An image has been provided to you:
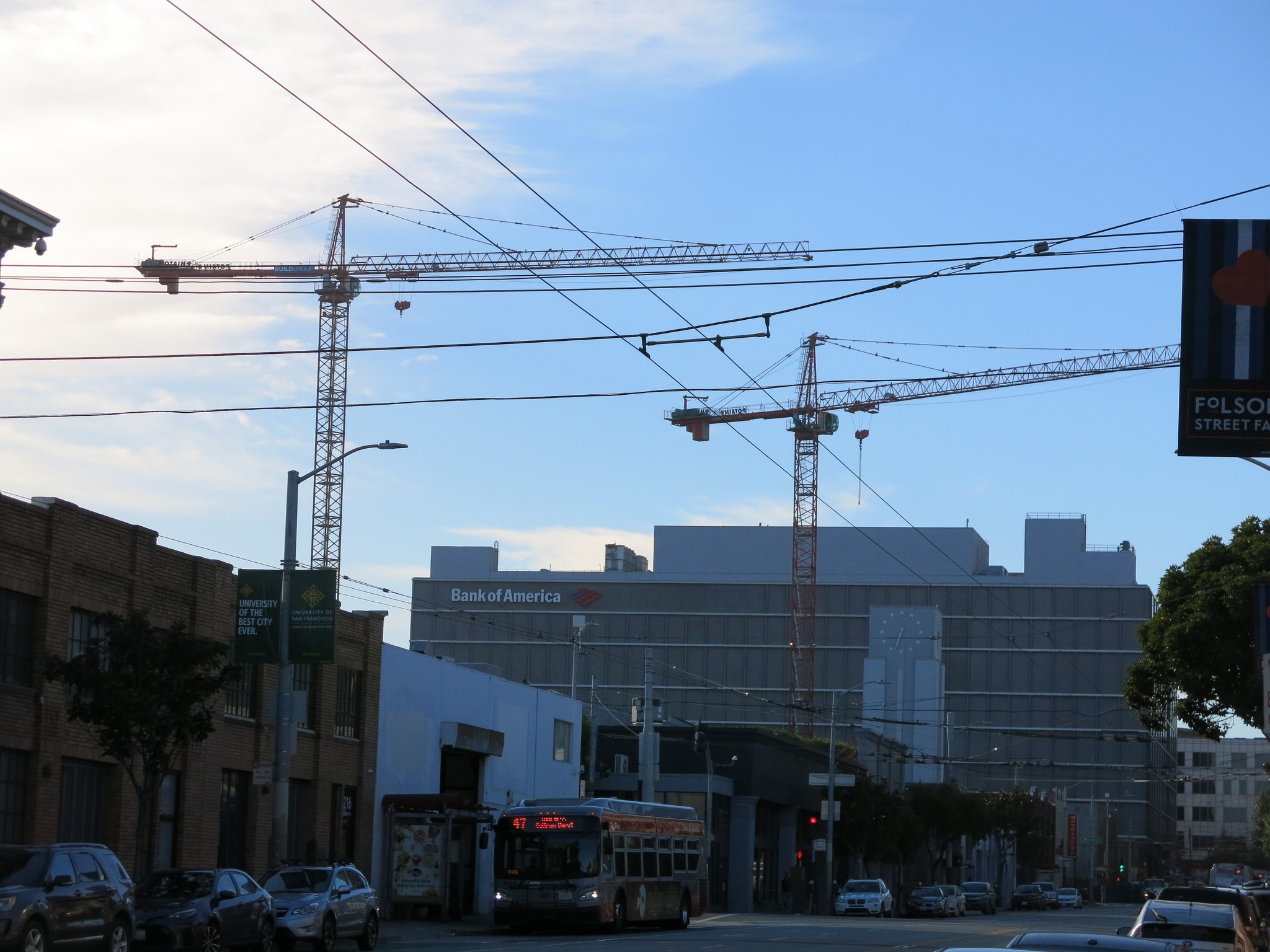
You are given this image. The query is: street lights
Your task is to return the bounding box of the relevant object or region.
[1060,778,1091,885]
[824,677,892,917]
[1103,794,1137,904]
[1013,758,1054,885]
[570,619,601,697]
[268,439,411,874]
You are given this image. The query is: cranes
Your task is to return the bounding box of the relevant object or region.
[667,339,1181,730]
[136,196,815,603]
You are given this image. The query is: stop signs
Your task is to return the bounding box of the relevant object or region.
[1060,857,1071,862]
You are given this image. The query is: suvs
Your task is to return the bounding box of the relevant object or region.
[1155,878,1270,952]
[834,877,894,915]
[1,843,138,952]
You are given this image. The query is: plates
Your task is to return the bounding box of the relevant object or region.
[422,853,434,866]
[413,829,427,843]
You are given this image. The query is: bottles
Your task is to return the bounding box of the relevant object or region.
[434,861,439,876]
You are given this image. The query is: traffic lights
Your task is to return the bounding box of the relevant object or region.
[810,812,819,831]
[1116,865,1124,883]
[796,850,808,861]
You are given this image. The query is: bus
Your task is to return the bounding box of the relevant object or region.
[478,797,709,932]
[256,859,380,952]
[1209,863,1254,887]
[1140,878,1173,903]
[1251,869,1270,883]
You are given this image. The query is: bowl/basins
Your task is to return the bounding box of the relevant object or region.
[412,868,422,876]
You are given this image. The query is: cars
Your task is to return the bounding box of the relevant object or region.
[1115,898,1265,952]
[135,869,277,952]
[932,883,967,918]
[1011,880,1088,913]
[1005,931,1187,952]
[956,881,996,916]
[905,886,948,918]
